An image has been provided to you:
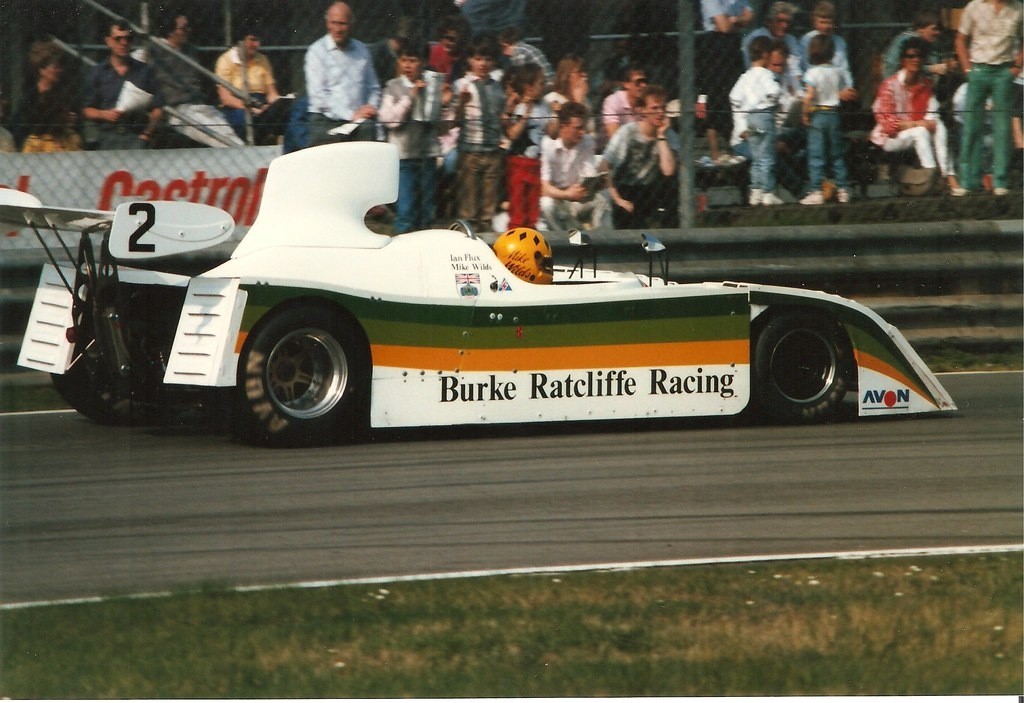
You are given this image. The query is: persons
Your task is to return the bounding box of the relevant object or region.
[493,227,553,284]
[1,0,1024,234]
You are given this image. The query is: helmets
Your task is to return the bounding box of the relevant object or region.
[493,226,554,284]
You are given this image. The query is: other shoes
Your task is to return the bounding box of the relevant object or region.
[992,187,1012,196]
[748,188,767,206]
[714,153,747,164]
[762,193,784,206]
[949,187,971,196]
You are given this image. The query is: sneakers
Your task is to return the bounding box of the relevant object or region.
[798,191,824,205]
[837,189,849,203]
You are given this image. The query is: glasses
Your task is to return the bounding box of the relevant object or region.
[439,33,459,43]
[107,34,133,43]
[628,77,649,86]
[904,53,924,59]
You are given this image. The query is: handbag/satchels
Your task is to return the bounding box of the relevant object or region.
[895,164,938,196]
